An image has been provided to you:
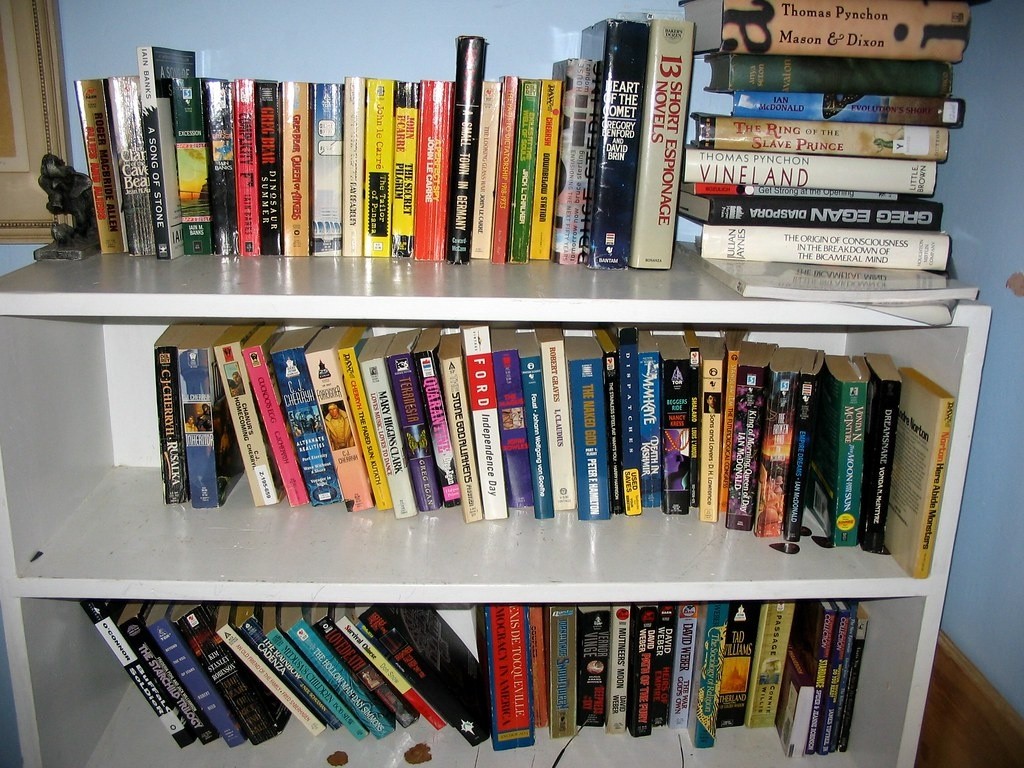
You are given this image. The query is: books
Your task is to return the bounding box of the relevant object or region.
[76,1,981,306]
[153,323,956,580]
[78,600,872,759]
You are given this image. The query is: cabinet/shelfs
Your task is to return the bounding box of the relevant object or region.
[0,260,996,768]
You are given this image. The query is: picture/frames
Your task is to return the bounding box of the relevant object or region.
[0,0,75,245]
